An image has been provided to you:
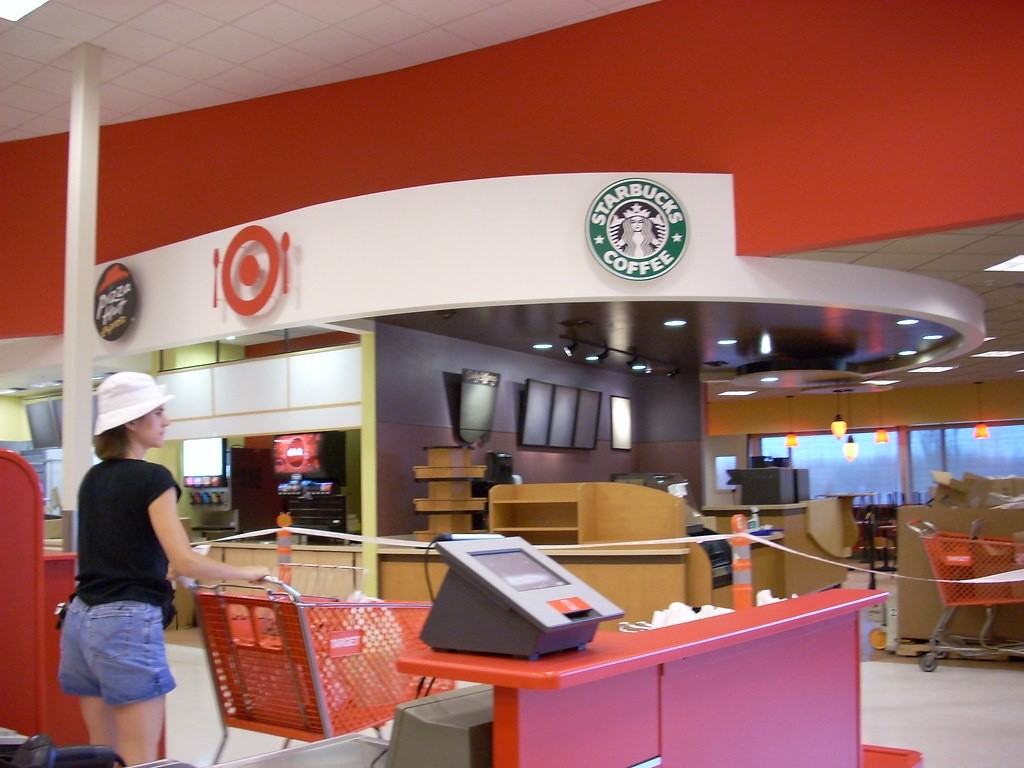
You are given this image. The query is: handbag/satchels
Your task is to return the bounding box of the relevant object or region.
[162,586,178,631]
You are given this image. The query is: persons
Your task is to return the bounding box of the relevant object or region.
[60,371,271,768]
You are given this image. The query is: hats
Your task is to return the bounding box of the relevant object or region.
[93,371,175,436]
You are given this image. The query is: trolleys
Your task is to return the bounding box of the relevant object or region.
[904,519,1023,671]
[190,562,454,767]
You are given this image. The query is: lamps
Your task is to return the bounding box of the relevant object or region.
[972,381,990,440]
[844,389,859,463]
[585,341,610,362]
[625,355,646,370]
[784,395,798,447]
[563,330,580,357]
[666,369,678,378]
[876,391,890,444]
[831,390,847,440]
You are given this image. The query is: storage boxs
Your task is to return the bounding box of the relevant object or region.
[931,470,1024,509]
